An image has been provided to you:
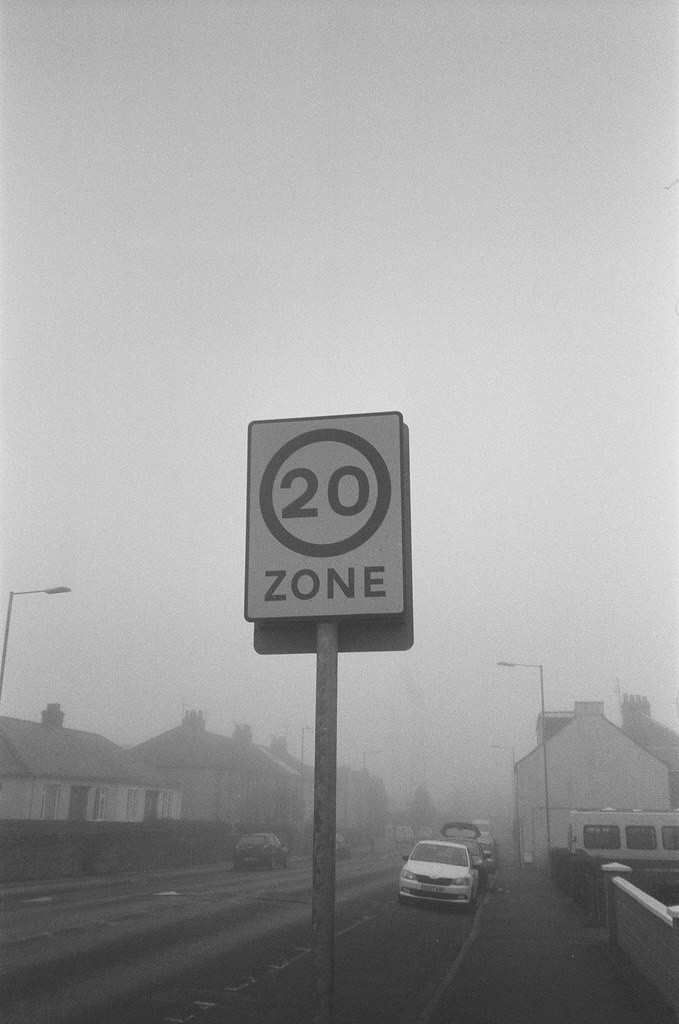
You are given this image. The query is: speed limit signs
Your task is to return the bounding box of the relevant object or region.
[259,425,395,558]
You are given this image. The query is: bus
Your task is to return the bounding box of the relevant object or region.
[566,807,679,868]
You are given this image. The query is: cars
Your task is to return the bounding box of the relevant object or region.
[231,832,290,873]
[429,818,498,878]
[396,839,479,911]
[305,832,350,861]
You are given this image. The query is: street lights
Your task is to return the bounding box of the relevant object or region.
[490,745,522,872]
[1,586,73,703]
[496,661,555,877]
